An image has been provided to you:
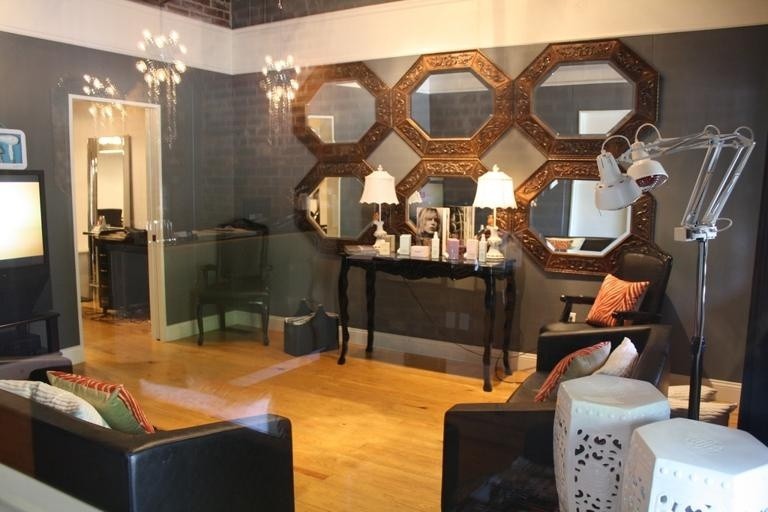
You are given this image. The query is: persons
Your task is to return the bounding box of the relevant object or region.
[413,206,438,238]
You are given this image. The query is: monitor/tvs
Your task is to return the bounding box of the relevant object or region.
[0,170,50,269]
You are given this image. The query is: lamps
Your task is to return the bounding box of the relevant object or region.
[472,166,519,258]
[593,112,757,417]
[360,165,399,245]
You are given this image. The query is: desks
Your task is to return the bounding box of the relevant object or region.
[332,246,518,391]
[92,230,148,317]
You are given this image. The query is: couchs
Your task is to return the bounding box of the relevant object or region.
[0,355,295,511]
[437,322,673,512]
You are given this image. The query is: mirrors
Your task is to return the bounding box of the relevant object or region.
[90,136,129,233]
[513,38,658,160]
[390,159,510,249]
[291,163,389,254]
[510,159,656,273]
[291,60,393,162]
[390,49,514,158]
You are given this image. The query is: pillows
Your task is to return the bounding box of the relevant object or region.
[531,336,610,400]
[0,376,114,430]
[45,367,154,436]
[590,336,638,379]
[585,267,649,326]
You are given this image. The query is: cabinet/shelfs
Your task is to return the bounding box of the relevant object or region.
[0,310,73,382]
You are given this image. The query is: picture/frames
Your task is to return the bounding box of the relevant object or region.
[0,127,28,171]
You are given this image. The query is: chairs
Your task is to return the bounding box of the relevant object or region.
[192,213,271,347]
[536,234,673,335]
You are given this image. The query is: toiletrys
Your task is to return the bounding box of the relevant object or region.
[479,233,487,263]
[478,234,488,262]
[465,239,479,260]
